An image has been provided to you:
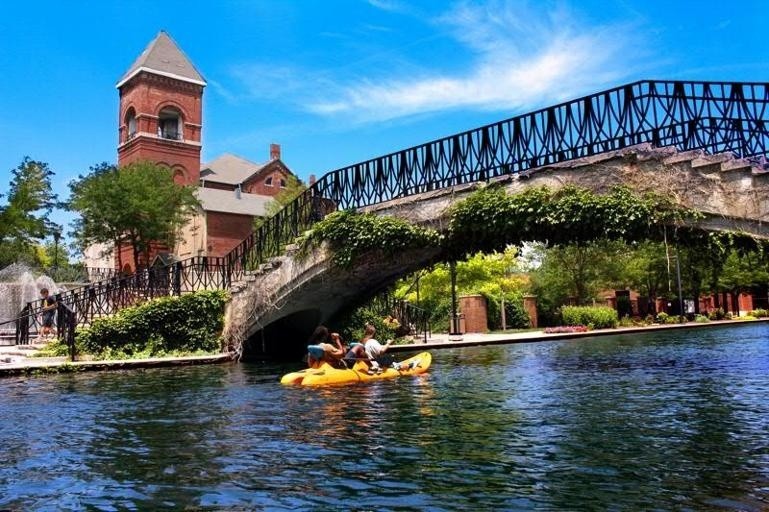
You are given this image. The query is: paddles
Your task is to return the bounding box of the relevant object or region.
[343,357,393,364]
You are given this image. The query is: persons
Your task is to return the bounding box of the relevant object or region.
[34,288,58,341]
[360,324,420,370]
[306,325,382,375]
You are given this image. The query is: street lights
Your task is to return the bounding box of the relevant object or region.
[53,227,62,271]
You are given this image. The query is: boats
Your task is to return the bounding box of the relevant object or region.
[279,350,434,388]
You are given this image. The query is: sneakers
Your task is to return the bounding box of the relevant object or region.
[412,359,421,369]
[369,367,379,371]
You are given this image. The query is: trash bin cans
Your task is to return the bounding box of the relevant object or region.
[449,313,466,335]
